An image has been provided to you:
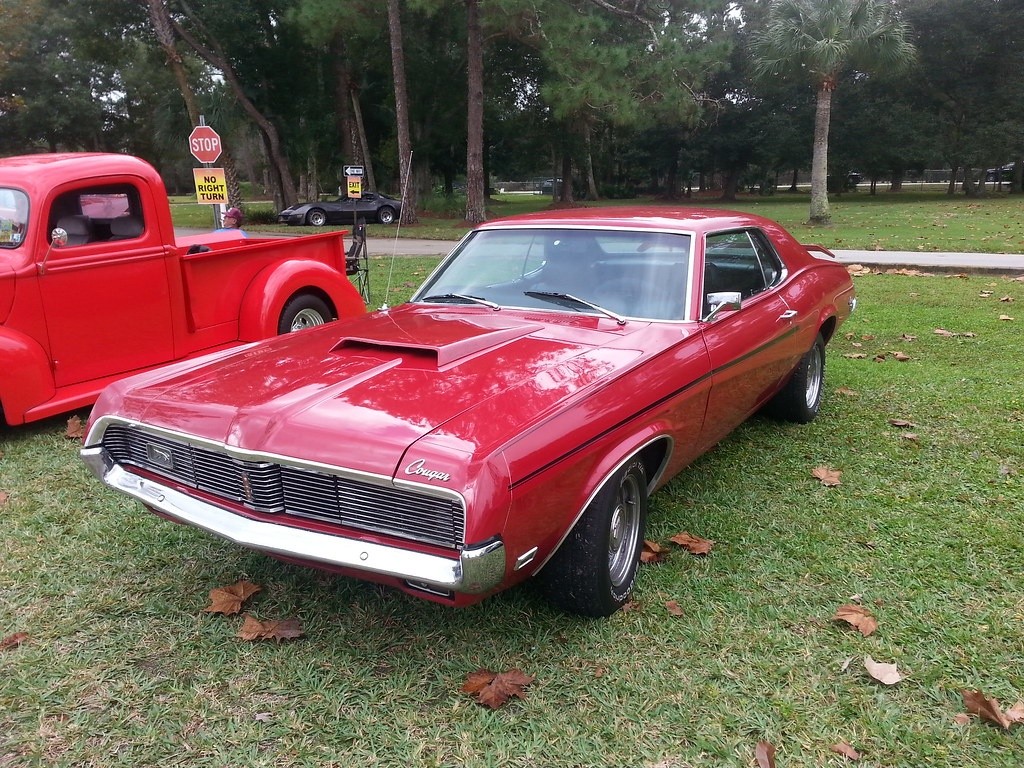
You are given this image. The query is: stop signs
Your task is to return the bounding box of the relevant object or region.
[188,125,223,164]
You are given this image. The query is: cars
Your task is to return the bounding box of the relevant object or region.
[984,161,1016,182]
[79,204,857,620]
[276,190,402,228]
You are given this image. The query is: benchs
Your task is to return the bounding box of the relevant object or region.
[602,259,773,301]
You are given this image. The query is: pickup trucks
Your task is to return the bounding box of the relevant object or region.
[1,151,367,435]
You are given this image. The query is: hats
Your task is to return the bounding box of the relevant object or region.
[222,208,242,219]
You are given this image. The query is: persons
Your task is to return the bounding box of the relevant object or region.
[211,207,248,237]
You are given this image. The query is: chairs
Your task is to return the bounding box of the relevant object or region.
[345,217,371,306]
[53,215,93,248]
[108,217,144,241]
